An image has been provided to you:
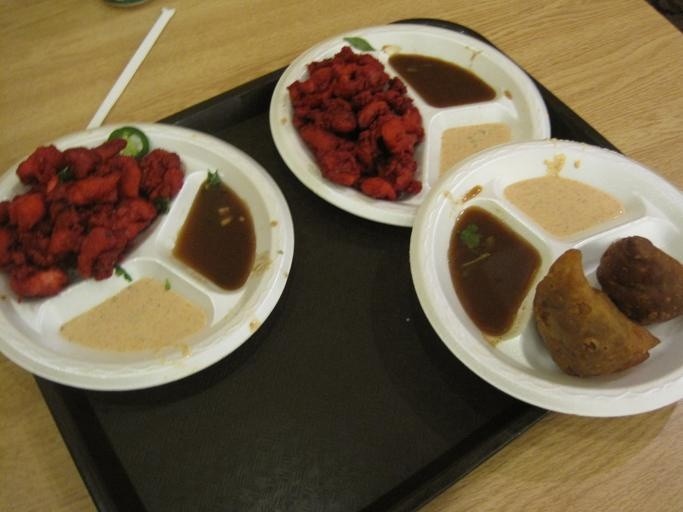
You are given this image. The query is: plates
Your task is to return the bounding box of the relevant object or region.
[0,115,294,392]
[24,12,657,512]
[418,136,683,409]
[277,15,543,226]
